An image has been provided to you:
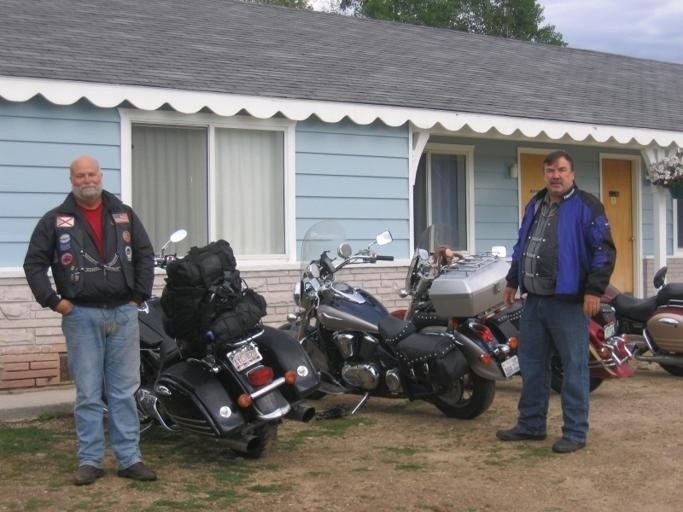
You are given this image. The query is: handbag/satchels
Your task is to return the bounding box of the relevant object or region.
[160,239,265,348]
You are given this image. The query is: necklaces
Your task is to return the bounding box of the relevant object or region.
[540,203,559,217]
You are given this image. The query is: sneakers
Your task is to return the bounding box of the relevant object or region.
[117,463,157,481]
[496,428,546,441]
[554,438,587,454]
[75,463,101,484]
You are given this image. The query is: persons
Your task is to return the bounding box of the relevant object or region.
[24,156,157,486]
[495,151,616,453]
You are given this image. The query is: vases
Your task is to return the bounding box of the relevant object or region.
[667,180,682,199]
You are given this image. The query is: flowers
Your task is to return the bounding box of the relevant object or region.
[646,146,683,184]
